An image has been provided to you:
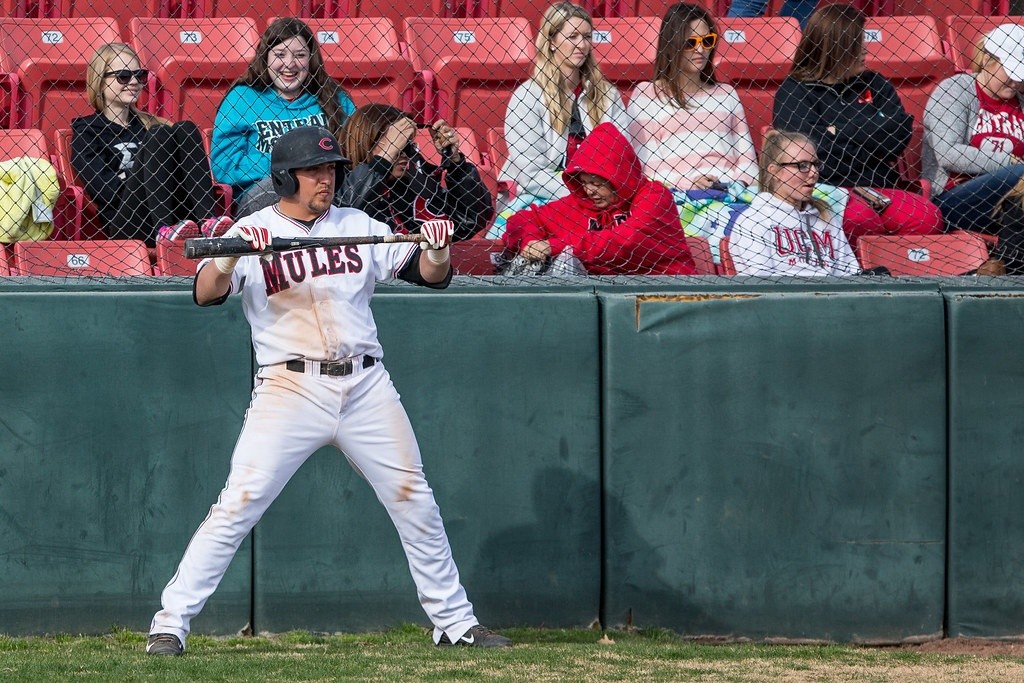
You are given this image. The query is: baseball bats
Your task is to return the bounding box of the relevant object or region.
[184,234,426,259]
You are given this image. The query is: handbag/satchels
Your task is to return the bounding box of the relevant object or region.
[503,246,588,276]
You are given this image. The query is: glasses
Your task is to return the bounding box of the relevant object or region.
[776,158,824,174]
[102,69,149,86]
[682,33,717,52]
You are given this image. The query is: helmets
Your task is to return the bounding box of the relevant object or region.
[272,126,350,198]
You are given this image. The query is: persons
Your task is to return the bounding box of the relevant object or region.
[328,104,493,241]
[496,4,630,200]
[627,4,759,191]
[920,24,1024,273]
[506,122,698,275]
[146,125,511,653]
[728,130,890,276]
[211,17,357,221]
[725,0,820,31]
[773,4,941,235]
[72,43,236,248]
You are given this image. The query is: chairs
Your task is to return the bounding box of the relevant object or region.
[0,0,1024,276]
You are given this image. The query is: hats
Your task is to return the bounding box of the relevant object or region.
[984,23,1024,81]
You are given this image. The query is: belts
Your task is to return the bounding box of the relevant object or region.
[286,354,379,377]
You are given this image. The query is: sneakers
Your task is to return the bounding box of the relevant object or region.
[155,220,198,244]
[199,217,234,237]
[146,633,184,657]
[434,620,510,647]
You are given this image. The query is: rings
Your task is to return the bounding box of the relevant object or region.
[448,132,452,137]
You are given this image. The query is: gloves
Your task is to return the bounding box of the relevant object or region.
[419,218,455,266]
[215,228,273,275]
